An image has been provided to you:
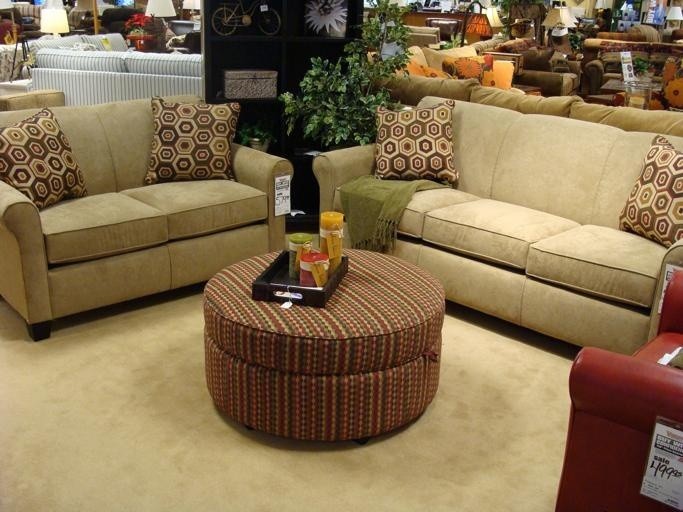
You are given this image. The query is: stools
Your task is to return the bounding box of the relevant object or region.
[204,246,447,446]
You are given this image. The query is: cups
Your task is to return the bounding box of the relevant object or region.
[623,80,652,112]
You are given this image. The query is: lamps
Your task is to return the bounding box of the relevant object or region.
[144,0,177,17]
[459,0,494,46]
[665,7,683,29]
[40,8,70,36]
[182,0,201,21]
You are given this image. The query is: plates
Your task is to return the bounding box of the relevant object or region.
[250,249,349,309]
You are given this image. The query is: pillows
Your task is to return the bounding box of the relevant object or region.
[519,49,555,72]
[407,58,458,81]
[0,108,86,210]
[442,54,496,88]
[494,39,529,53]
[619,133,683,248]
[145,95,240,187]
[372,101,456,186]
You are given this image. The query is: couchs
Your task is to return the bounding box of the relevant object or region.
[554,269,683,512]
[311,97,683,358]
[30,47,207,107]
[584,48,667,97]
[0,93,295,343]
[471,38,582,96]
[408,45,515,90]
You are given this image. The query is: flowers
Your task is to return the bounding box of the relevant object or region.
[650,57,683,112]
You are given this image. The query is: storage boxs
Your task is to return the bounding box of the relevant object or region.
[223,69,278,98]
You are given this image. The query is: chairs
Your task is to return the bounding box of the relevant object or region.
[438,45,477,57]
